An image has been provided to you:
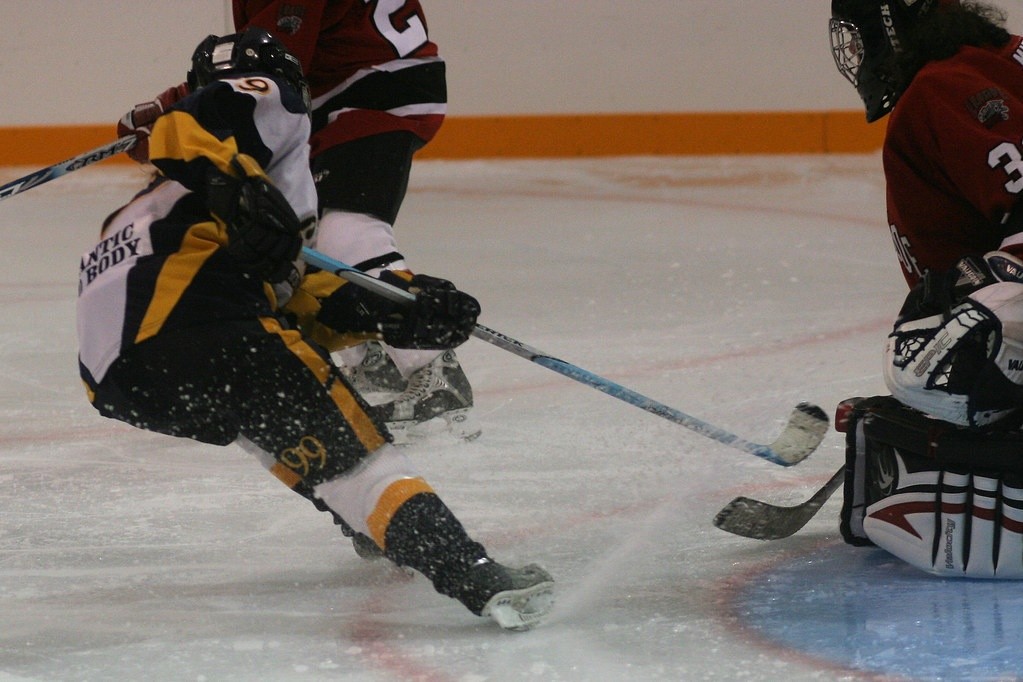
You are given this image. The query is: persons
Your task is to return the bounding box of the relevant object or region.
[73,28,558,631]
[826,0,1023,584]
[233,0,484,448]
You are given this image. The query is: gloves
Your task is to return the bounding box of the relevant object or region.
[207,154,301,286]
[372,269,482,350]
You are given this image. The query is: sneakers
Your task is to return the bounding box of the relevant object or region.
[337,342,409,403]
[457,555,560,631]
[373,349,482,449]
[350,534,409,581]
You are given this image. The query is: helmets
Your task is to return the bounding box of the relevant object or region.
[185,24,303,112]
[830,0,942,123]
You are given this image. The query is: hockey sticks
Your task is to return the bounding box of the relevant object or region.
[296,246,830,468]
[0,135,137,201]
[711,463,846,541]
